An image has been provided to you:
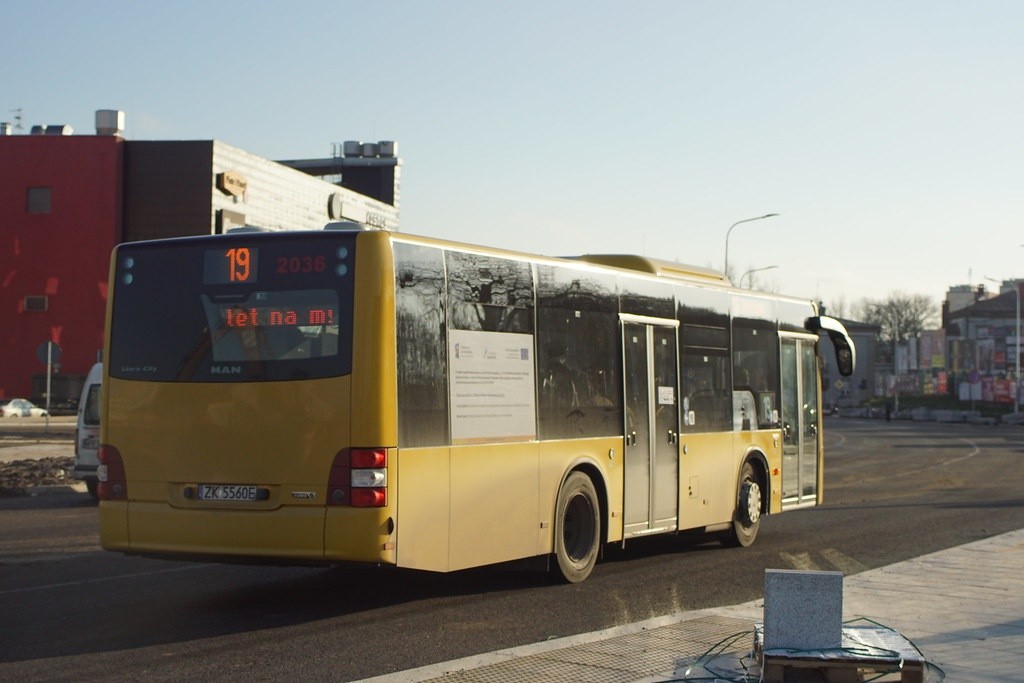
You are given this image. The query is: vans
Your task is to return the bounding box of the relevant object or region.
[72,362,104,501]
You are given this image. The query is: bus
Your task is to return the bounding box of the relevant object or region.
[99,221,858,582]
